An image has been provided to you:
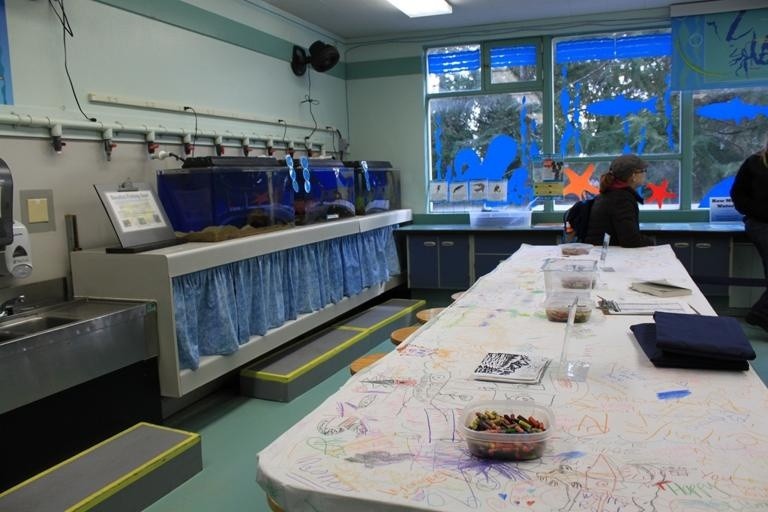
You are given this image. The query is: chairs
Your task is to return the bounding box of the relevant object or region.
[563,197,594,241]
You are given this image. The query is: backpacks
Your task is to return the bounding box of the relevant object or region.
[560,195,598,243]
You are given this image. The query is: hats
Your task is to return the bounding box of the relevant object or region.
[609,154,650,176]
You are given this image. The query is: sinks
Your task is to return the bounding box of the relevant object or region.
[0,316,80,346]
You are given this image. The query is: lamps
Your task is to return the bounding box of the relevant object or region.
[290,38,343,78]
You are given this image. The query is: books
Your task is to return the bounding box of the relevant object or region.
[630,278,693,297]
[473,352,553,384]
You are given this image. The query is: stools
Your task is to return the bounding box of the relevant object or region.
[349,288,469,376]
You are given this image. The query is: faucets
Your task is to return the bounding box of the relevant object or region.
[0,294,25,314]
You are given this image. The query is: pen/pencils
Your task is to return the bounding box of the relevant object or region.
[467,409,547,461]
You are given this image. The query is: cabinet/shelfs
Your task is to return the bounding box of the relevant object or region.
[401,233,745,294]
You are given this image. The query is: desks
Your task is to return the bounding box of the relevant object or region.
[256,239,765,510]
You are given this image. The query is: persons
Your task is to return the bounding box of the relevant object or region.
[730,132,768,332]
[544,159,553,169]
[584,154,656,248]
